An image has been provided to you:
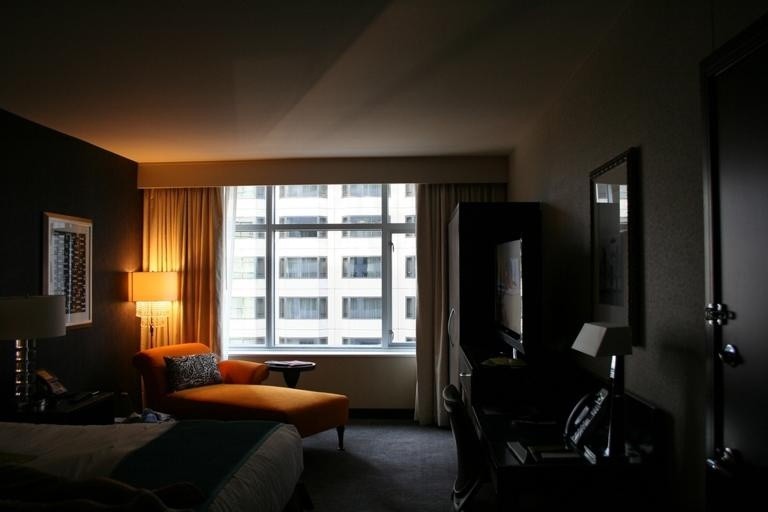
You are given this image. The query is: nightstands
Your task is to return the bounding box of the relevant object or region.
[10,389,117,423]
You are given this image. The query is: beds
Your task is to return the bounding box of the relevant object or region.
[1,421,310,511]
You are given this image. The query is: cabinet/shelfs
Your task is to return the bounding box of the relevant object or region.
[447,201,542,395]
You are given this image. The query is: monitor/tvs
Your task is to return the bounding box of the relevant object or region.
[486,236,535,354]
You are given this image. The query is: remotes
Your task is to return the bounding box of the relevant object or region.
[67,390,93,404]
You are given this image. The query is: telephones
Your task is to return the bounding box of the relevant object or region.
[564,388,611,450]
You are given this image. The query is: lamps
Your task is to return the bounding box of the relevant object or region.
[0,291,67,409]
[126,270,180,348]
[570,322,634,458]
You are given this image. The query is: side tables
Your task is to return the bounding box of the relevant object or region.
[265,360,317,387]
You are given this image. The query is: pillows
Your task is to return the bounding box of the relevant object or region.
[162,352,223,392]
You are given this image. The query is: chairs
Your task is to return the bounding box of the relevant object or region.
[442,385,490,512]
[132,341,348,451]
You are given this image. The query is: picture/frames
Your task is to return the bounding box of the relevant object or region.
[43,212,94,332]
[589,146,643,347]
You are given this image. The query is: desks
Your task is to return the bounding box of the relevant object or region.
[470,406,641,497]
